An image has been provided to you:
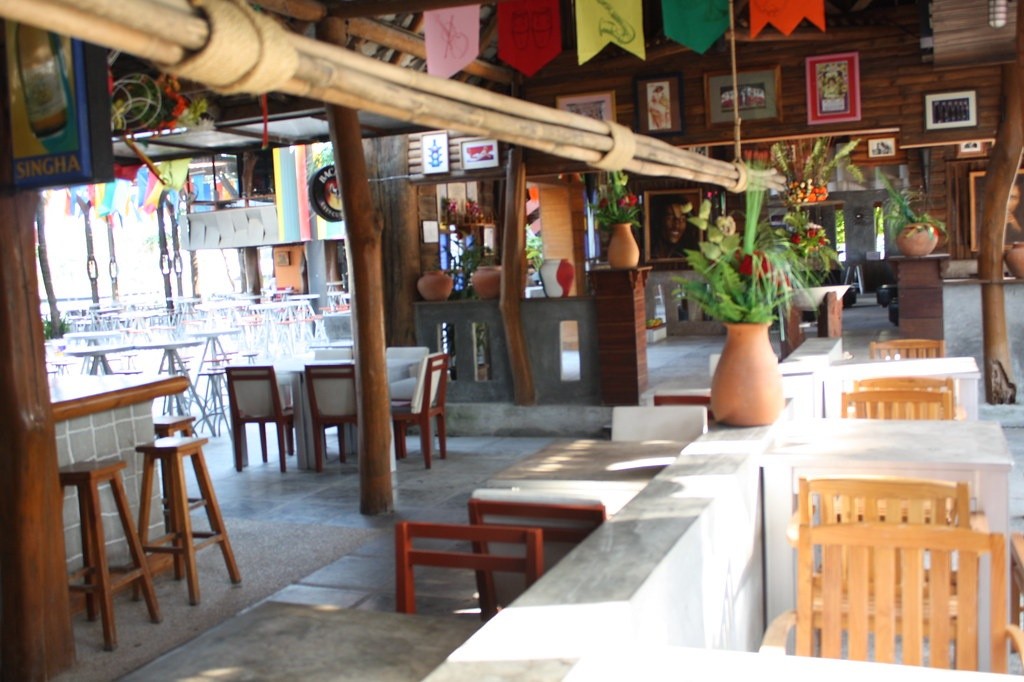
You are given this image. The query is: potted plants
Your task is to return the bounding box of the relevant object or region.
[869,168,951,257]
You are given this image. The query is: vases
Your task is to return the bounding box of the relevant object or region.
[711,320,789,427]
[607,222,639,270]
[792,285,852,312]
[1005,241,1024,280]
[472,265,503,299]
[417,270,454,302]
[539,257,576,299]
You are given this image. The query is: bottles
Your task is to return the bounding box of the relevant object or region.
[16,22,68,138]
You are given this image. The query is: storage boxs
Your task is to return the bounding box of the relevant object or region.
[646,325,667,344]
[866,252,880,261]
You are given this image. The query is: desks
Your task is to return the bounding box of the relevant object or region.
[114,600,484,682]
[271,356,424,469]
[48,376,187,616]
[469,432,694,517]
[117,308,157,343]
[286,293,321,318]
[188,327,242,438]
[63,343,135,377]
[193,299,252,329]
[818,354,981,423]
[134,340,216,439]
[251,300,310,357]
[62,330,121,376]
[760,415,1018,676]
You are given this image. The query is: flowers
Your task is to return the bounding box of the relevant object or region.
[113,67,205,132]
[585,178,835,328]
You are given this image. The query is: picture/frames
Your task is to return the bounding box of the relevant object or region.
[866,135,898,161]
[703,63,785,131]
[641,188,707,267]
[805,50,861,126]
[922,86,984,135]
[420,130,451,176]
[275,251,291,267]
[970,169,1024,253]
[554,89,619,126]
[633,71,687,138]
[459,136,502,172]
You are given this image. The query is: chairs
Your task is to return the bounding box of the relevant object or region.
[225,347,727,620]
[757,339,1024,676]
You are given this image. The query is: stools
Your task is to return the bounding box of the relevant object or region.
[844,261,866,296]
[44,280,353,654]
